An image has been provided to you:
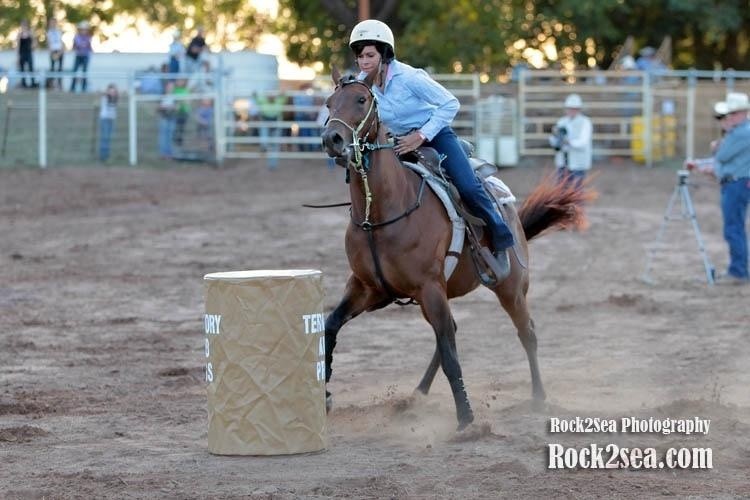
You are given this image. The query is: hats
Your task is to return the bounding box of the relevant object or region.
[714,93,749,119]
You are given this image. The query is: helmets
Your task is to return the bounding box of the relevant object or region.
[565,94,582,110]
[348,19,395,58]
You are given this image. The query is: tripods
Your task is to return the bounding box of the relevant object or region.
[642,171,717,289]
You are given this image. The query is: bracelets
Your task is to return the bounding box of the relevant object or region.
[420,133,426,140]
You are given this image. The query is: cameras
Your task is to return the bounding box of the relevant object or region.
[558,126,569,137]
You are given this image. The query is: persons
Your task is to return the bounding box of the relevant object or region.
[348,20,516,278]
[45,18,66,90]
[548,94,593,190]
[155,37,216,159]
[15,20,40,87]
[99,83,118,164]
[252,83,324,169]
[710,92,750,281]
[69,21,92,92]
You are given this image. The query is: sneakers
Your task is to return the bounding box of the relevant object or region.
[716,272,736,283]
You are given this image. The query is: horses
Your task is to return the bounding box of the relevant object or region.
[320,62,604,440]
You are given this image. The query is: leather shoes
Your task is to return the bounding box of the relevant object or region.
[488,250,511,279]
